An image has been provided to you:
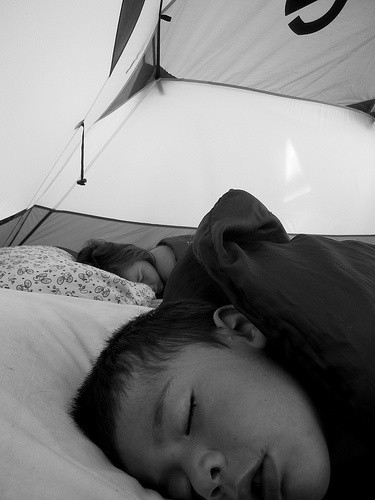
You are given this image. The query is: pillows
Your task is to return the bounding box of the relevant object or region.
[0,244,158,310]
[0,287,164,500]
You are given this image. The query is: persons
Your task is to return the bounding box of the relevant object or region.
[63,284,332,500]
[74,232,191,296]
